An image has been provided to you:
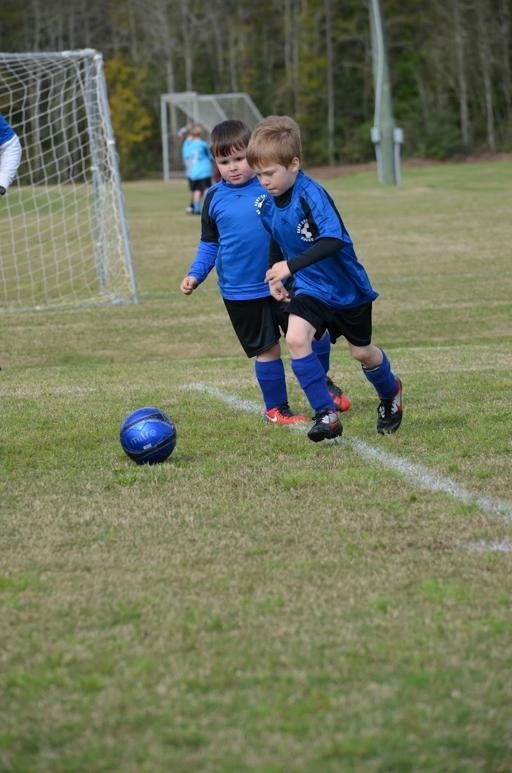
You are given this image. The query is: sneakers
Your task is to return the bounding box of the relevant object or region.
[377,377,402,435]
[188,206,201,215]
[264,378,349,442]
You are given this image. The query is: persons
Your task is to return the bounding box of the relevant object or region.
[177,117,222,214]
[246,116,404,439]
[180,120,350,425]
[0,112,22,199]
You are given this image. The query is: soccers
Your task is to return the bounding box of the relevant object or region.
[119,408,175,465]
